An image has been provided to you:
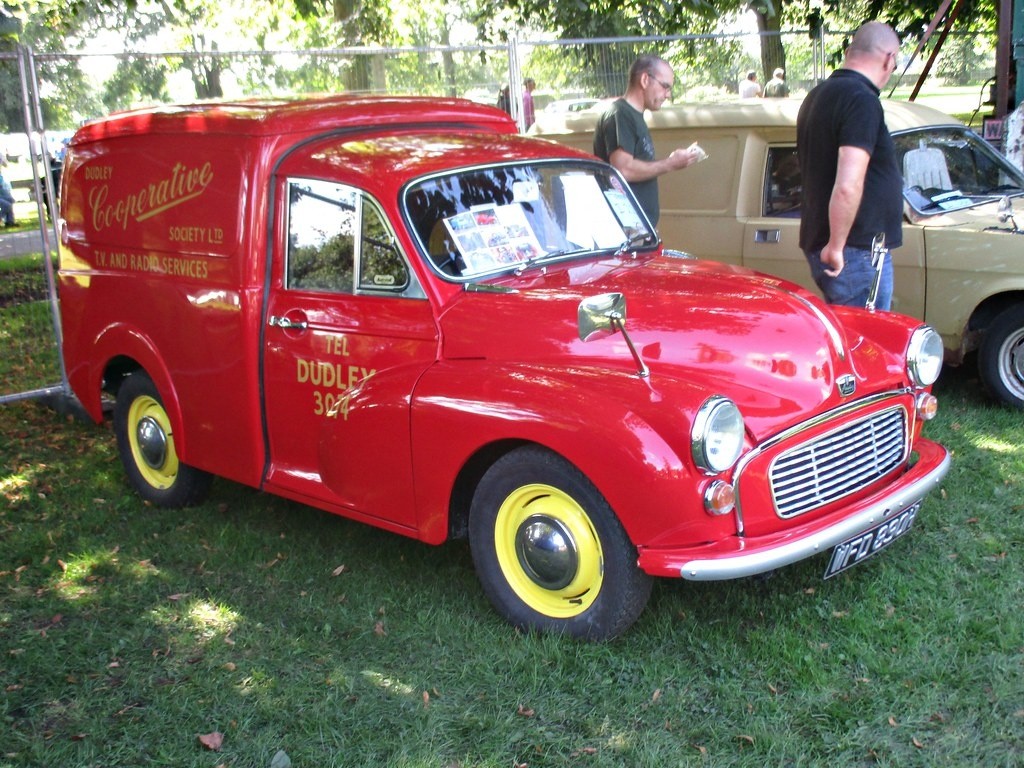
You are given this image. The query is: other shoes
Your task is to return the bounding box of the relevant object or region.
[5,222,20,228]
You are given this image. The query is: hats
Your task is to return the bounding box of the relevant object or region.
[774,68,784,77]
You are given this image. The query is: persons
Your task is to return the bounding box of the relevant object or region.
[738,69,760,98]
[593,55,700,232]
[797,21,904,312]
[494,77,536,133]
[761,67,791,98]
[0,154,21,227]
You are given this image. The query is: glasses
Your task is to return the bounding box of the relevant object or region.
[888,53,898,73]
[648,73,670,90]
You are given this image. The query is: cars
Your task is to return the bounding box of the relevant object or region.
[51,101,951,652]
[524,95,1024,411]
